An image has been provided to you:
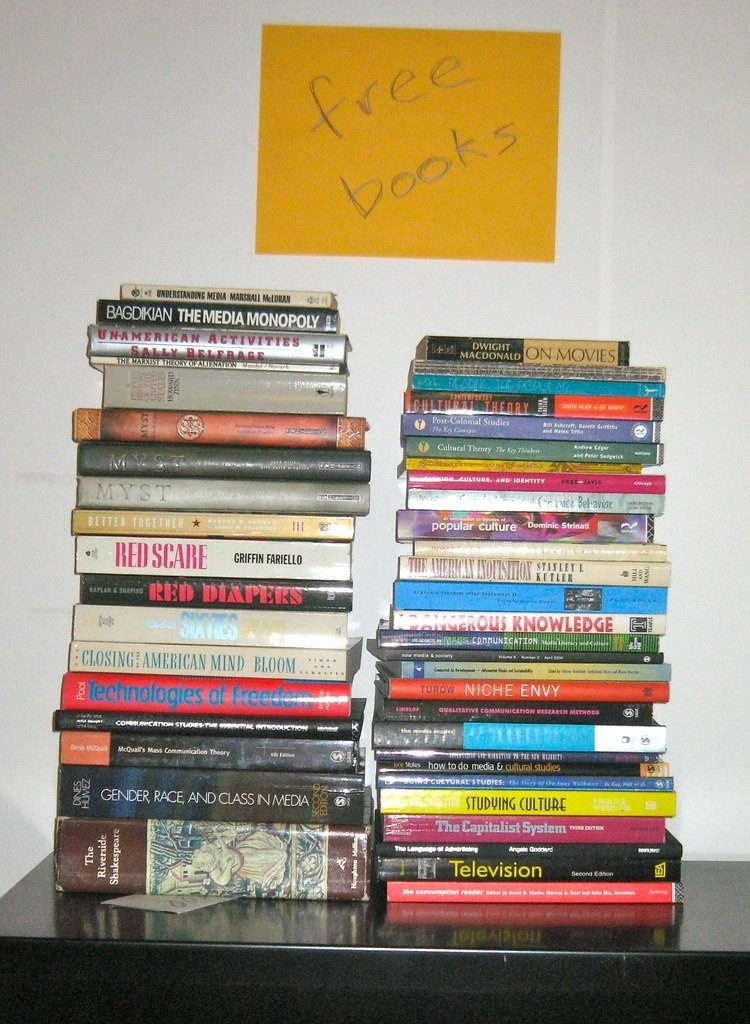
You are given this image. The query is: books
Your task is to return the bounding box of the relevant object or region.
[51,283,378,902]
[365,334,685,905]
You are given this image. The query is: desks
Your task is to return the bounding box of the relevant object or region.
[0,851,750,1024]
